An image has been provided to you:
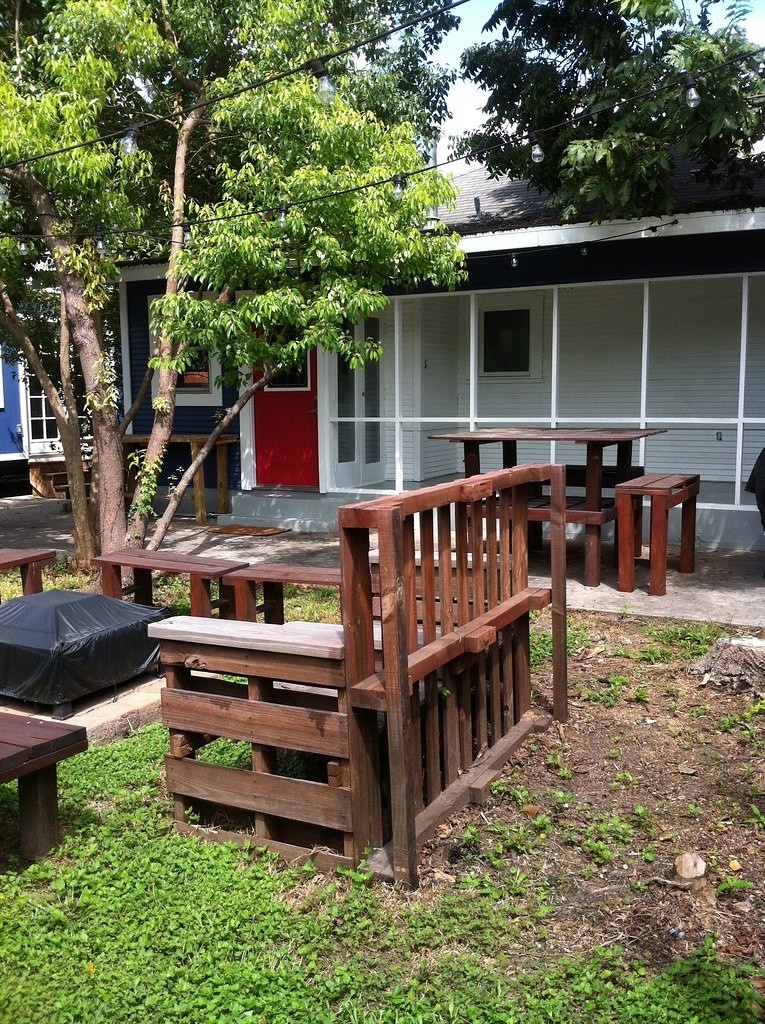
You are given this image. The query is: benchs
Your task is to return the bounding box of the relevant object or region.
[1,547,57,599]
[91,547,250,622]
[148,462,568,882]
[0,711,89,861]
[223,564,342,627]
[463,465,700,597]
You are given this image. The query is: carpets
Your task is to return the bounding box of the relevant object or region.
[208,524,293,536]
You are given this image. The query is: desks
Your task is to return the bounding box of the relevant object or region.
[427,428,668,587]
[80,434,239,526]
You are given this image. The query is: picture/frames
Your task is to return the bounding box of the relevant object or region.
[263,349,311,392]
